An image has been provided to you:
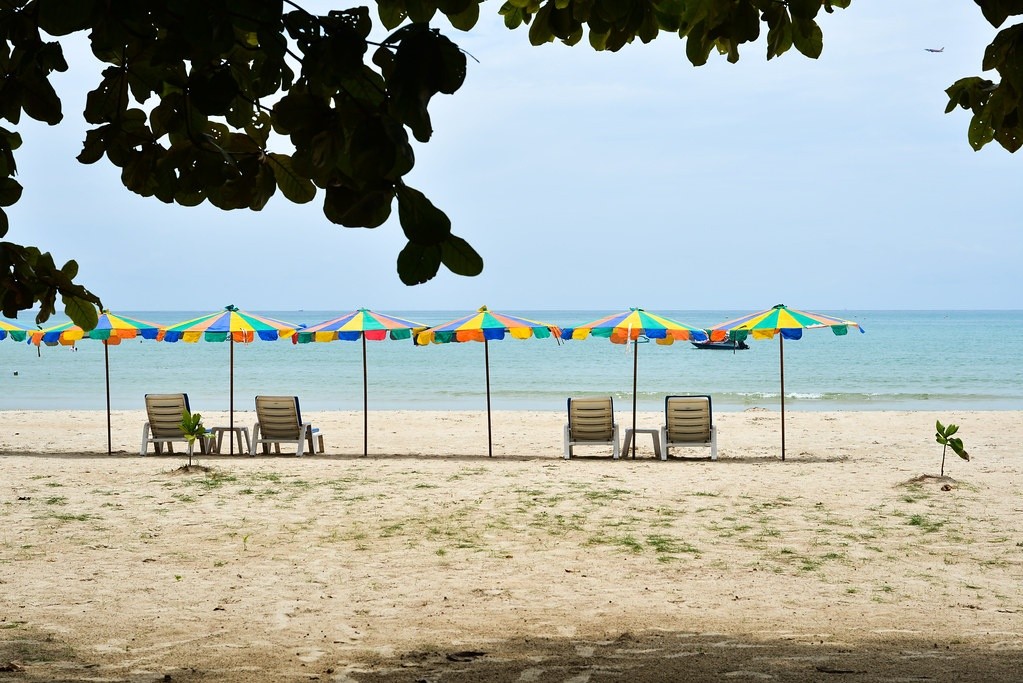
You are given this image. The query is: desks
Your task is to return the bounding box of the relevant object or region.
[207,426,251,456]
[621,426,661,459]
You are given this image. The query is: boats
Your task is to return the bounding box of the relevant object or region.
[690,333,750,350]
[298,309,303,312]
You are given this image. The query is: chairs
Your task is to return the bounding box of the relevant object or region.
[139,392,212,457]
[661,395,717,461]
[563,396,620,460]
[250,395,324,457]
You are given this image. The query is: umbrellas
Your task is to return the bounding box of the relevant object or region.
[414,305,565,458]
[27,308,167,457]
[154,304,307,457]
[0,318,40,343]
[702,304,865,462]
[292,307,433,457]
[561,307,709,461]
